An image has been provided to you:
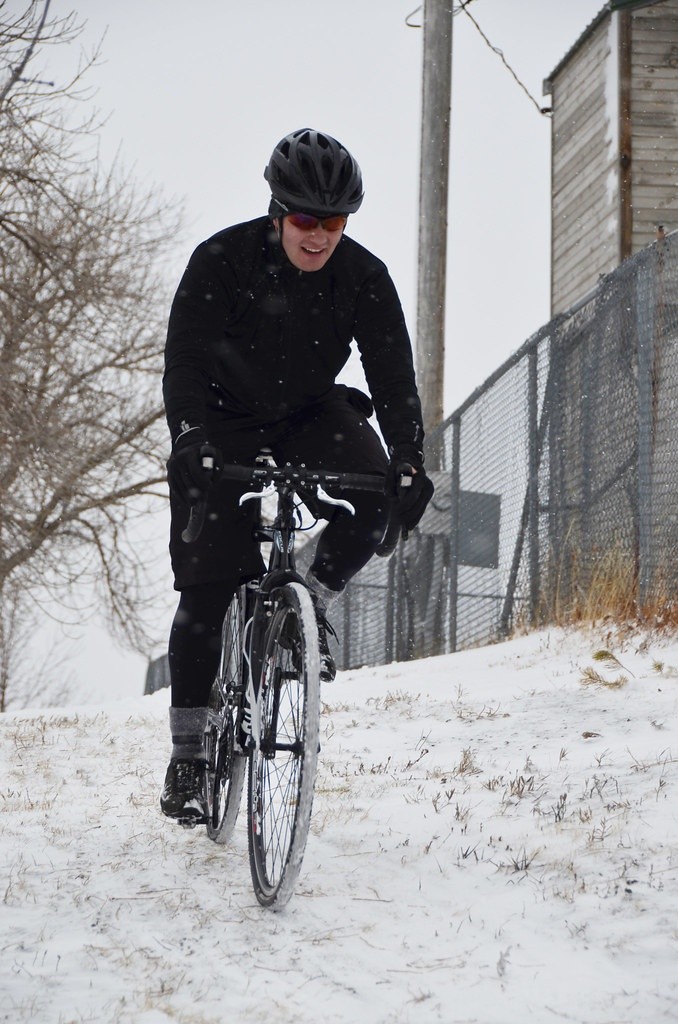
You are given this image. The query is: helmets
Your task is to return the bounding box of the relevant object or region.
[268,128,364,215]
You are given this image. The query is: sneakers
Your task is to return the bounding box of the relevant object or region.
[279,604,340,682]
[159,758,206,824]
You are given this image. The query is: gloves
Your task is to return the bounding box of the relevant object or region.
[166,431,223,503]
[383,456,436,528]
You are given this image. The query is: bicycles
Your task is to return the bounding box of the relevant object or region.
[169,438,418,911]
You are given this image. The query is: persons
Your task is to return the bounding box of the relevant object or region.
[159,129,434,815]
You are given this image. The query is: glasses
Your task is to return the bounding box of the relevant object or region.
[272,198,348,232]
